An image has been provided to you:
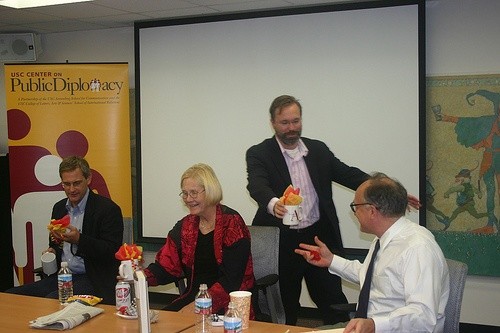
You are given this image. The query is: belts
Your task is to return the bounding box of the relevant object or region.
[282,221,321,233]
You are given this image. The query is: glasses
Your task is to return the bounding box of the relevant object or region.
[61,178,86,189]
[350,202,372,212]
[179,190,206,198]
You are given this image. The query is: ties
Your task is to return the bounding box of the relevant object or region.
[355,239,380,318]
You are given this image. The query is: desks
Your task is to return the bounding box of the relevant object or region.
[179,318,319,333]
[0,293,208,333]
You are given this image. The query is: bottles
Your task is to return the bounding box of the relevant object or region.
[57,261,73,306]
[194,284,213,333]
[223,301,243,333]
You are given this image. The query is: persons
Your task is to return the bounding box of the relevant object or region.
[292,171,449,333]
[243,95,422,330]
[1,155,124,306]
[116,162,256,326]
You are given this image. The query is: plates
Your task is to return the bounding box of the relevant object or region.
[116,311,138,319]
[206,316,223,326]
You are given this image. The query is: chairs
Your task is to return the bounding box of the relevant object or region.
[330,259,468,333]
[175,226,287,325]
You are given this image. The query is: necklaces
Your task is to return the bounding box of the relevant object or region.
[199,224,214,231]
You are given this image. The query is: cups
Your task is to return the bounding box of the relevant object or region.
[282,204,305,225]
[41,251,57,275]
[229,291,252,330]
[119,258,139,281]
